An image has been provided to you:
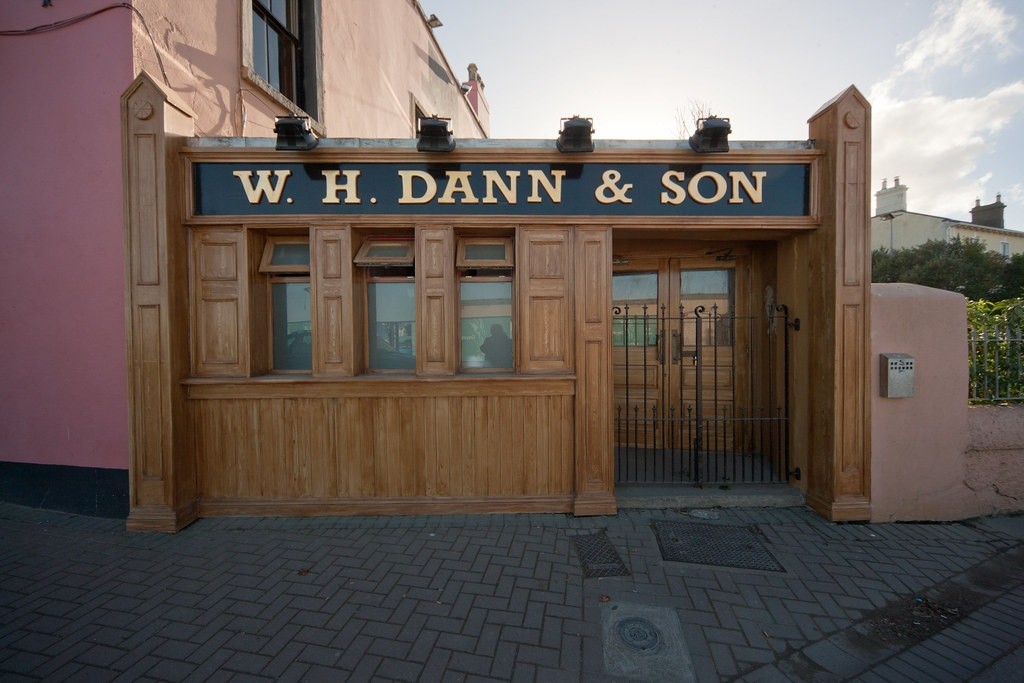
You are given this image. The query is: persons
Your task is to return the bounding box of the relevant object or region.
[480,324,512,368]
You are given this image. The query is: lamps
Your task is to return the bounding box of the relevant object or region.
[416,114,457,153]
[556,114,595,152]
[459,83,472,96]
[687,116,731,152]
[272,110,318,151]
[427,13,443,29]
